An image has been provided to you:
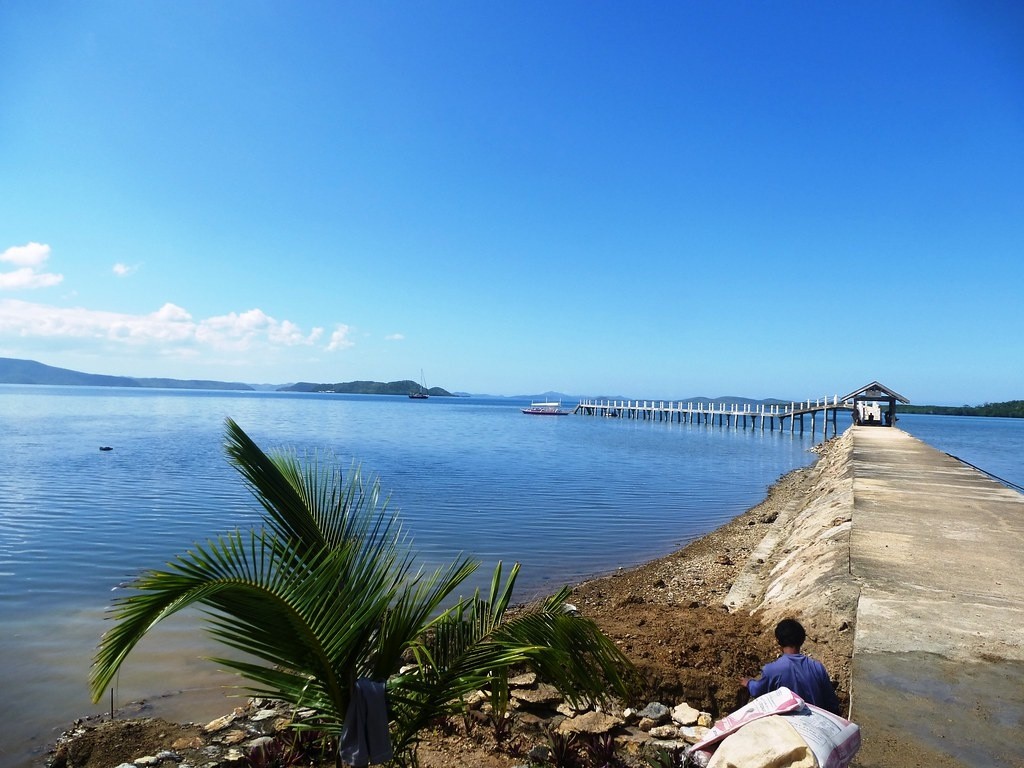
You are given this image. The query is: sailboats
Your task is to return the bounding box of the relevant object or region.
[408,368,430,398]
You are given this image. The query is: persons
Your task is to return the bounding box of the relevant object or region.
[739,619,840,714]
[869,413,873,424]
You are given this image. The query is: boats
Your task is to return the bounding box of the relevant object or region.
[520,407,569,415]
